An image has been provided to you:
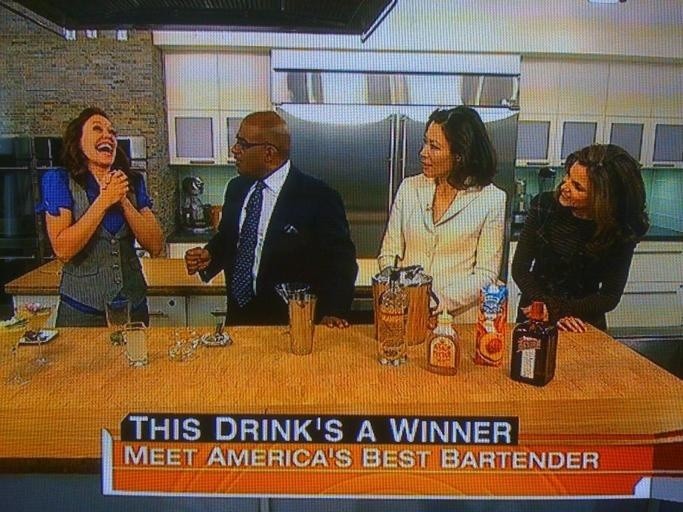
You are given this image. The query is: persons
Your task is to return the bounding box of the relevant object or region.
[510,144,648,336]
[185,112,357,328]
[41,106,165,326]
[378,105,506,327]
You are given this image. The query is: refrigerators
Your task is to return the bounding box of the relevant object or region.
[268,46,521,319]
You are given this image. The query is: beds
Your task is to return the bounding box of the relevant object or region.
[0,316,683,465]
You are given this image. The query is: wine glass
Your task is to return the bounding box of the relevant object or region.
[14,300,60,367]
[273,280,312,337]
[2,321,33,387]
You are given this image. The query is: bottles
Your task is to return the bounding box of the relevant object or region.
[376,289,410,366]
[508,301,560,386]
[166,327,206,365]
[425,304,463,377]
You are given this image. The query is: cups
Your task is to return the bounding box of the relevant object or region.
[287,292,318,358]
[121,319,153,369]
[102,287,133,347]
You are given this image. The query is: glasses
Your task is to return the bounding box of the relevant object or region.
[239,139,279,151]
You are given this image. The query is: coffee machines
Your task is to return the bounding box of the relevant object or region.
[178,175,212,233]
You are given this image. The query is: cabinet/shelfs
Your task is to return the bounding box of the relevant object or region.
[606,61,682,122]
[605,240,683,327]
[166,50,272,112]
[520,59,608,122]
[169,112,266,165]
[14,295,63,328]
[147,297,229,328]
[517,115,601,167]
[605,117,682,170]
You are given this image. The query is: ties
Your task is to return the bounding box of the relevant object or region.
[231,180,267,309]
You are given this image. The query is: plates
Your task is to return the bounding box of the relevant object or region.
[16,328,60,346]
